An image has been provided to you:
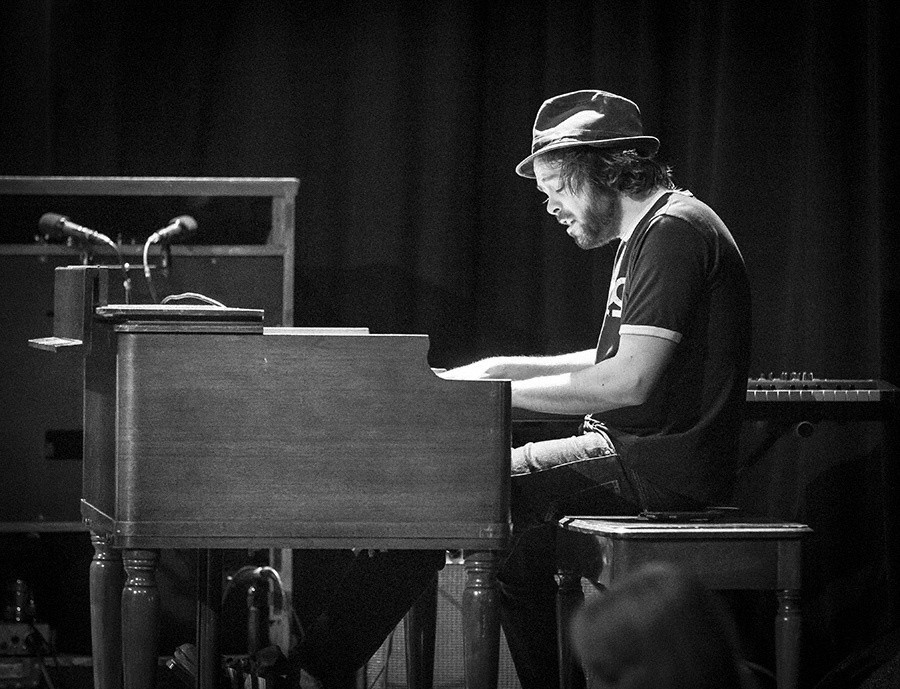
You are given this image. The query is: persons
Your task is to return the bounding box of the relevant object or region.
[571,561,779,689]
[228,89,753,689]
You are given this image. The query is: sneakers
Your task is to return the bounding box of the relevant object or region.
[174,644,292,689]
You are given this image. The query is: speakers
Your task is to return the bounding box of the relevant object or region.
[357,564,521,688]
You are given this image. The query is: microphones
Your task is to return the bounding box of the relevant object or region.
[148,215,198,243]
[39,214,110,244]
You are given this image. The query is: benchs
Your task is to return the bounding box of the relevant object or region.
[555,515,813,689]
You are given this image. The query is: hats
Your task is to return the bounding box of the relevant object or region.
[515,89,660,179]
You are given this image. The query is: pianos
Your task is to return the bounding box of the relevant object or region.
[746,366,899,689]
[28,305,515,688]
